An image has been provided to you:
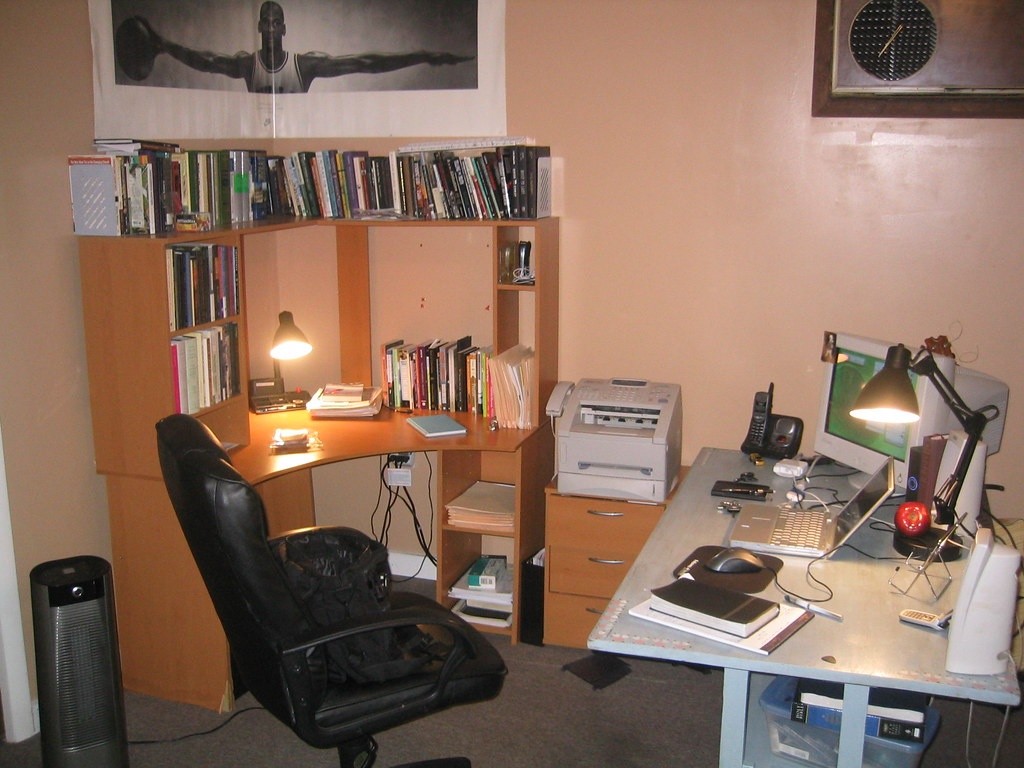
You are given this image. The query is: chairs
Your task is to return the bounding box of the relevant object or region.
[153,414,507,768]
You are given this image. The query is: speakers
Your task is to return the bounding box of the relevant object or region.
[945,528,1021,676]
[933,430,987,536]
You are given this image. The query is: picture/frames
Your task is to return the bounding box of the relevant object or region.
[812,0,1024,119]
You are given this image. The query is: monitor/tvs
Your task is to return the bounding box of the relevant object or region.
[813,332,1009,498]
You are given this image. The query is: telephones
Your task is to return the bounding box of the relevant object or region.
[740,382,804,459]
[545,381,576,416]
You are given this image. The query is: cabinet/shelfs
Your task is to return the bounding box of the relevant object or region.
[74,215,560,713]
[542,464,692,649]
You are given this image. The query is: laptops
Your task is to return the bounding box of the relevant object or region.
[729,456,894,559]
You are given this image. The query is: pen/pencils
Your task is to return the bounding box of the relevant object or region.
[721,488,775,493]
[785,595,843,621]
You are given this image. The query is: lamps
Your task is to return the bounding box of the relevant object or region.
[271,311,313,401]
[850,343,987,556]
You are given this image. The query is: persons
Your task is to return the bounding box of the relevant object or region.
[135,0,477,94]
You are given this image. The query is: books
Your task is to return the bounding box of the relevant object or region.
[445,480,515,532]
[267,150,394,218]
[380,335,496,418]
[487,343,535,429]
[171,322,241,414]
[68,138,273,235]
[406,414,468,437]
[628,572,816,655]
[649,577,781,639]
[306,382,383,416]
[797,678,926,725]
[448,561,513,606]
[166,242,240,331]
[451,599,512,627]
[389,146,551,219]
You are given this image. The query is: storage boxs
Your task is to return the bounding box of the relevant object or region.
[758,676,939,768]
[468,558,505,589]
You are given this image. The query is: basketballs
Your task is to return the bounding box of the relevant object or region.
[115,18,156,80]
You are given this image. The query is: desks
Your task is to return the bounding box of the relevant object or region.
[586,447,1021,768]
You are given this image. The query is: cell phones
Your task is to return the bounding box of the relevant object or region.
[899,608,953,631]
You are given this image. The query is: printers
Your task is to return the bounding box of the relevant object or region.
[545,377,683,501]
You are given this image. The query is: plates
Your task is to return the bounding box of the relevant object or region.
[267,429,322,450]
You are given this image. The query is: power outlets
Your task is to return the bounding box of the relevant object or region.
[389,453,414,466]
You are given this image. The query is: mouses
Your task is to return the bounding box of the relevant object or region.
[705,548,763,573]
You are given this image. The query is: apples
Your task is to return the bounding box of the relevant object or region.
[895,501,930,537]
